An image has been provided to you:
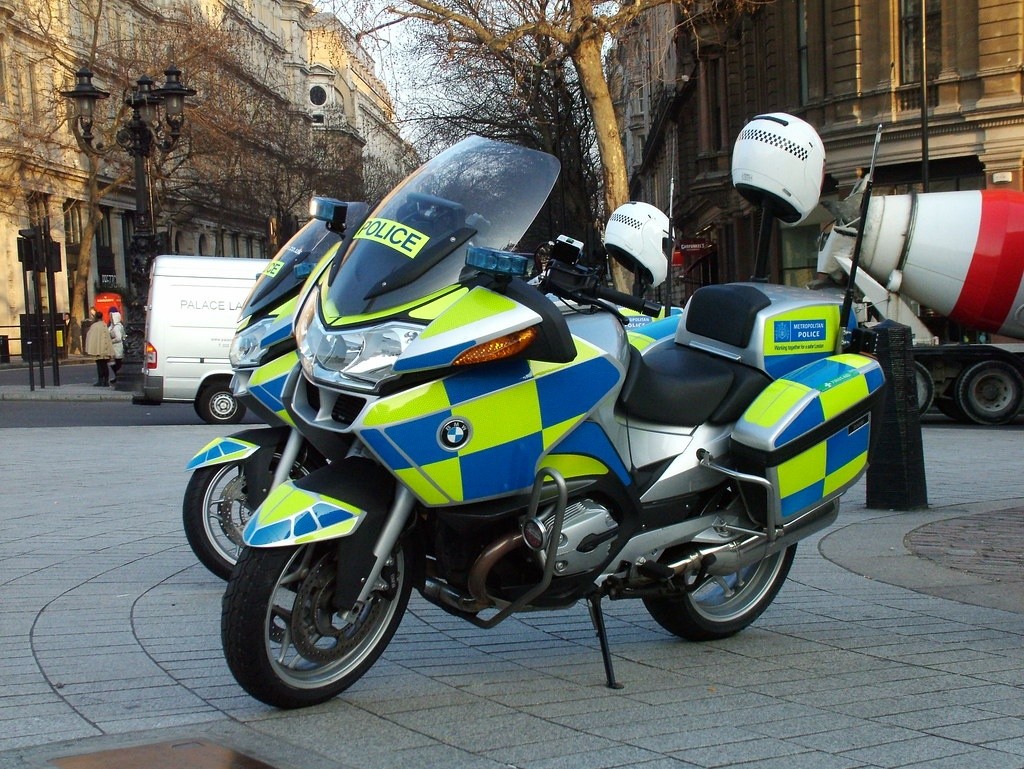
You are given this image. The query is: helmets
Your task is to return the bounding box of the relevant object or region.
[604,201,675,289]
[732,113,826,227]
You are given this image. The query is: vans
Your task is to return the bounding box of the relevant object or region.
[128,254,272,425]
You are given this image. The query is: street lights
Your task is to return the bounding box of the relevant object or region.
[59,60,199,394]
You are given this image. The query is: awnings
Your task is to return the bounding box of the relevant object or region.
[680,238,716,273]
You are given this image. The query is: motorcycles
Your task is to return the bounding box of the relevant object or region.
[177,132,888,709]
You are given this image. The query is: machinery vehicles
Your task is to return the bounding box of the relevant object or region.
[807,173,1024,429]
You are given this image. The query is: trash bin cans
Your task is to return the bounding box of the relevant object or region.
[81,319,93,355]
[0,335,11,363]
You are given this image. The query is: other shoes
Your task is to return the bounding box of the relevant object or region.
[93,382,109,387]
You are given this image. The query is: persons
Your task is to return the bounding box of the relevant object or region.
[84,312,114,387]
[107,311,126,383]
[63,313,71,337]
